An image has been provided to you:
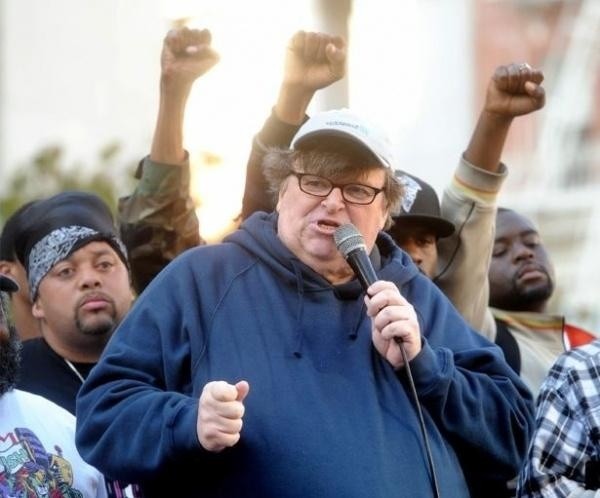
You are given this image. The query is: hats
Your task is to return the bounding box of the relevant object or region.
[0,272,21,292]
[390,169,455,239]
[289,108,396,170]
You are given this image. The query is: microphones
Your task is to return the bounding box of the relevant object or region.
[332,222,404,344]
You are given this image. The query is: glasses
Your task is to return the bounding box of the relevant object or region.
[287,170,386,205]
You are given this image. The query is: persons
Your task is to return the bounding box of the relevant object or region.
[514,333,600,498]
[423,60,596,497]
[0,269,110,496]
[1,12,224,343]
[12,219,142,497]
[240,25,456,289]
[76,107,540,498]
